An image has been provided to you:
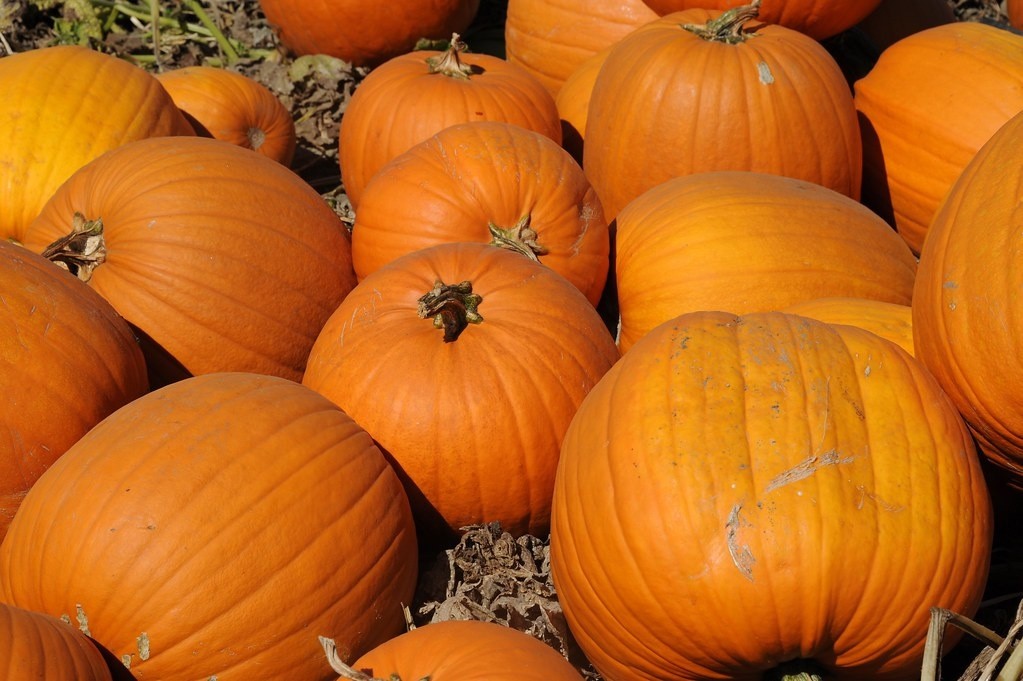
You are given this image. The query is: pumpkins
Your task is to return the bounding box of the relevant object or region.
[0,0,1023,681]
